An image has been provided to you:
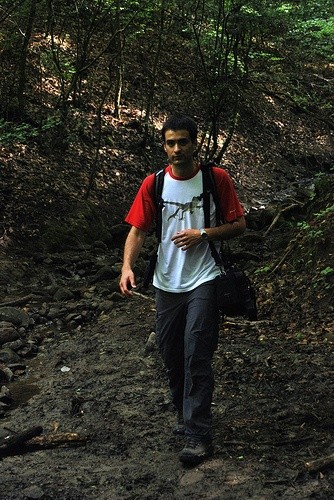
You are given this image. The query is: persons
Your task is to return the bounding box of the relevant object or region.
[119,116,246,462]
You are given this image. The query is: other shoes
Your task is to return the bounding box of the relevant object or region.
[176,406,186,433]
[178,435,212,464]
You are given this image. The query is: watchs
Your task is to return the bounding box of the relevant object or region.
[199,228,208,241]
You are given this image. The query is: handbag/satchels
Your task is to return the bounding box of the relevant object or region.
[217,265,258,321]
